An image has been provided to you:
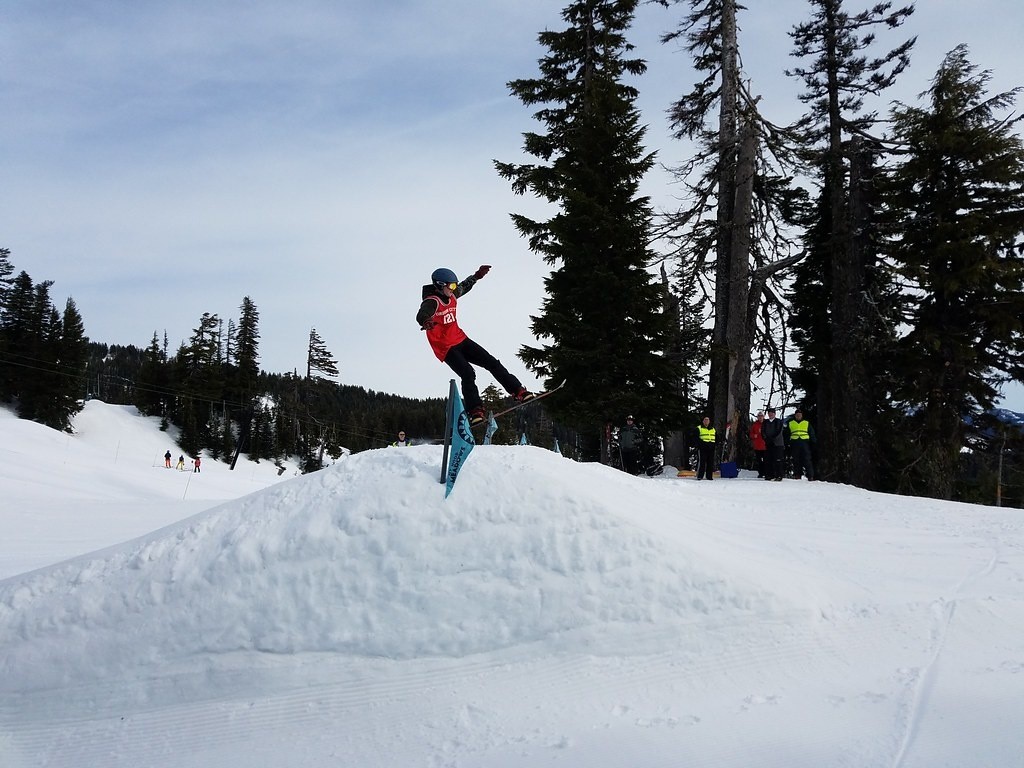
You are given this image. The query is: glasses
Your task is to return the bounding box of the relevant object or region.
[400,434,406,436]
[627,418,634,421]
[434,281,457,290]
[758,415,763,417]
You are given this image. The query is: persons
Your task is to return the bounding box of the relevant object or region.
[416,265,537,426]
[750,408,784,481]
[783,410,816,481]
[164,450,171,468]
[176,455,184,470]
[393,431,411,447]
[194,457,200,473]
[692,416,720,481]
[619,413,643,475]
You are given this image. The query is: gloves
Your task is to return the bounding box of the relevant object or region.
[420,318,440,331]
[475,265,491,279]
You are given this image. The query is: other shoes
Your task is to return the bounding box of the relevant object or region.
[765,476,771,480]
[697,477,702,480]
[707,478,713,480]
[774,476,782,482]
[808,476,814,481]
[758,474,763,478]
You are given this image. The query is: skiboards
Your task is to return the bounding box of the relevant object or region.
[163,465,173,469]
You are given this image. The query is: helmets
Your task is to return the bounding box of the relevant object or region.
[626,415,634,419]
[431,268,458,286]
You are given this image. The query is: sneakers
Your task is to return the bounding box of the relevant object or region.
[468,404,487,425]
[511,386,534,403]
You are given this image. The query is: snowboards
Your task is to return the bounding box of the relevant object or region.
[469,378,566,429]
